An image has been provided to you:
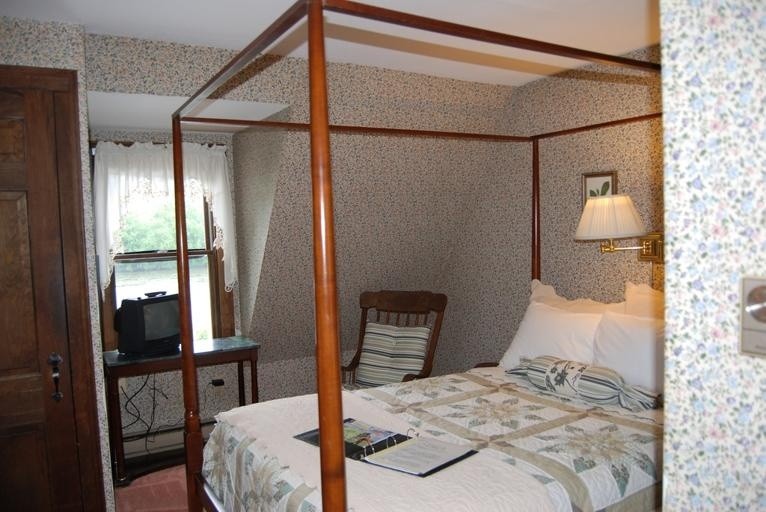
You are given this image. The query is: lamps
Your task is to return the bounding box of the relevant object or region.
[574,195,663,265]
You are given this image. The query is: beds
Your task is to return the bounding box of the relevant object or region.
[184,365,664,510]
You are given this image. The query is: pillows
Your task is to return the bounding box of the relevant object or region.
[498,297,664,409]
[355,321,429,384]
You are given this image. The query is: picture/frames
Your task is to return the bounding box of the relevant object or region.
[580,172,618,212]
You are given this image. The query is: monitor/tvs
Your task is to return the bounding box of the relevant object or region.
[114,294,181,359]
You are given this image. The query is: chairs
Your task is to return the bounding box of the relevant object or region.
[339,289,450,388]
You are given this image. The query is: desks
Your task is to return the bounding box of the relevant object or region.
[102,336,262,486]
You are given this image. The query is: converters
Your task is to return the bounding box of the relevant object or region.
[211,378,225,387]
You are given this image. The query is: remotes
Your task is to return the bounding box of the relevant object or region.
[145,290,167,296]
